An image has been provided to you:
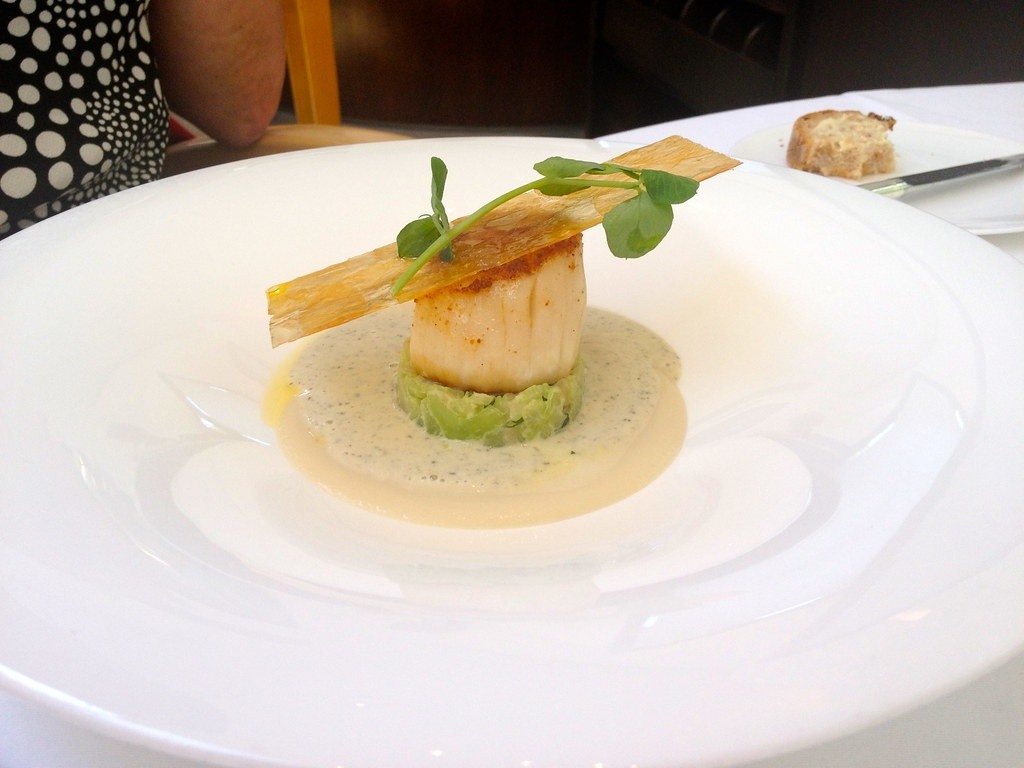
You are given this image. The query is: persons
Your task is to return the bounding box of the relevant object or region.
[1,1,289,251]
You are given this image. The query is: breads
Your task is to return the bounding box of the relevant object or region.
[787,110,897,180]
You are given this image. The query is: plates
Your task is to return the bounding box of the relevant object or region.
[0,136,1024,768]
[731,123,1024,238]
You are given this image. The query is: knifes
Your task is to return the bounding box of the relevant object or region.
[856,151,1024,198]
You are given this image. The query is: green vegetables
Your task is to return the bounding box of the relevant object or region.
[385,153,698,295]
